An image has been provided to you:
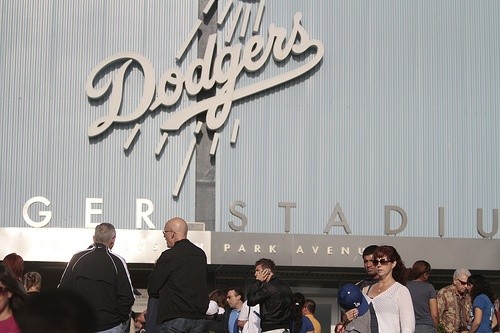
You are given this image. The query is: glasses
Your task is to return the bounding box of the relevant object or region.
[373,259,392,266]
[163,231,176,235]
[458,279,467,285]
[0,287,8,294]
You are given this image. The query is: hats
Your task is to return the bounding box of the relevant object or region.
[336,284,369,316]
[206,300,225,315]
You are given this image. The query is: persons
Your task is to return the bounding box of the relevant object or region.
[0,253,56,333]
[335,245,500,333]
[130,217,210,333]
[57,223,135,333]
[203,258,320,333]
